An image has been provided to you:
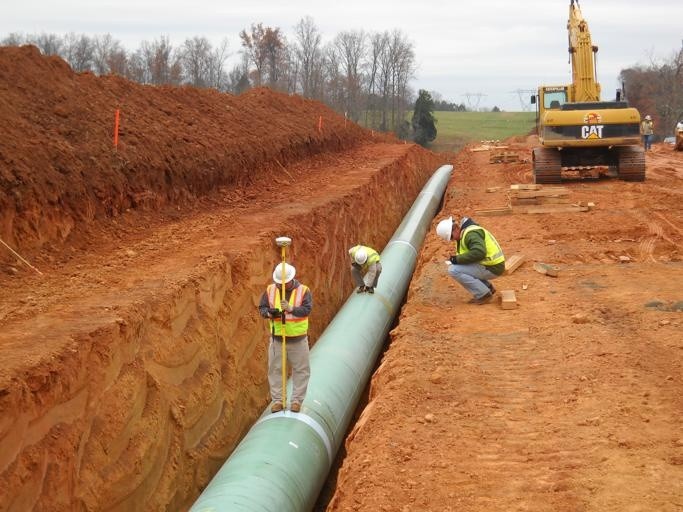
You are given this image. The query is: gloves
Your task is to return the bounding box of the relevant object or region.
[449,255,456,265]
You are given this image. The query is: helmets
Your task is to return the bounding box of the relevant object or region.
[435,215,452,244]
[272,263,297,285]
[353,248,368,265]
[644,115,651,121]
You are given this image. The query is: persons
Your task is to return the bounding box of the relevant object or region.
[259,260,311,414]
[346,243,382,294]
[435,216,505,306]
[639,115,654,152]
[676,120,682,131]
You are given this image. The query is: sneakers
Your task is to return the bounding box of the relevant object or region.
[290,403,300,412]
[270,402,285,413]
[356,286,374,294]
[467,284,497,304]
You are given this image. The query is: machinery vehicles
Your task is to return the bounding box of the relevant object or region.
[531,0,645,182]
